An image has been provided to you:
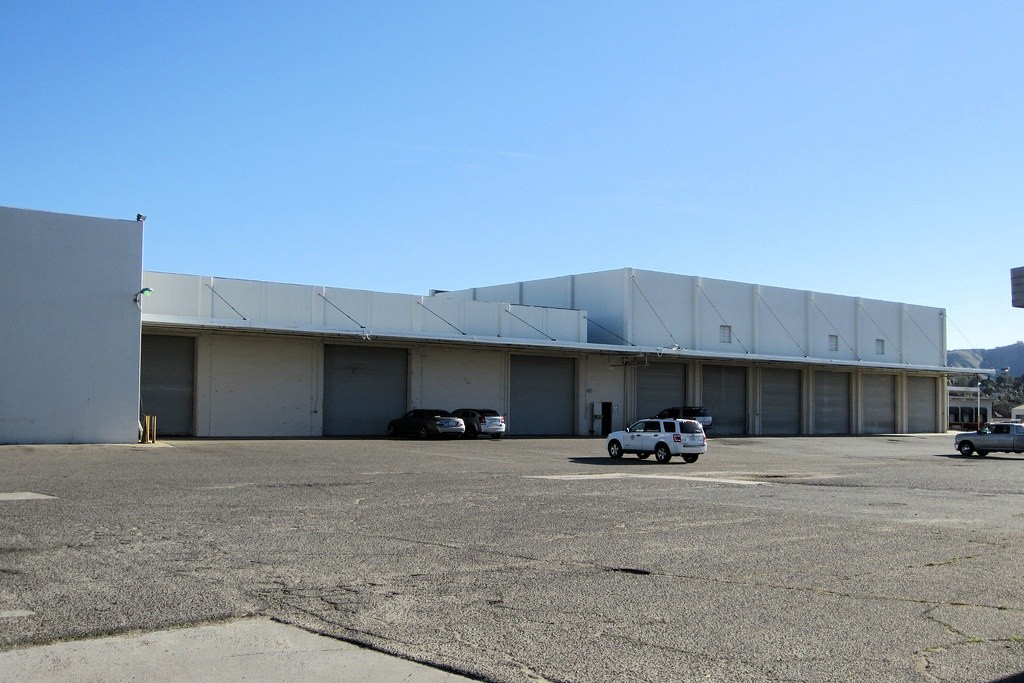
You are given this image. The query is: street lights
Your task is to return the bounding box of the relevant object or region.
[978,367,1010,430]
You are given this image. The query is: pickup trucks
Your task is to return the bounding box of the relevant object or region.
[954,422,1024,457]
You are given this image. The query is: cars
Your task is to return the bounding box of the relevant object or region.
[450,407,506,438]
[387,410,465,440]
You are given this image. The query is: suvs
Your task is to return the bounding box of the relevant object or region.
[650,406,713,433]
[606,417,709,463]
